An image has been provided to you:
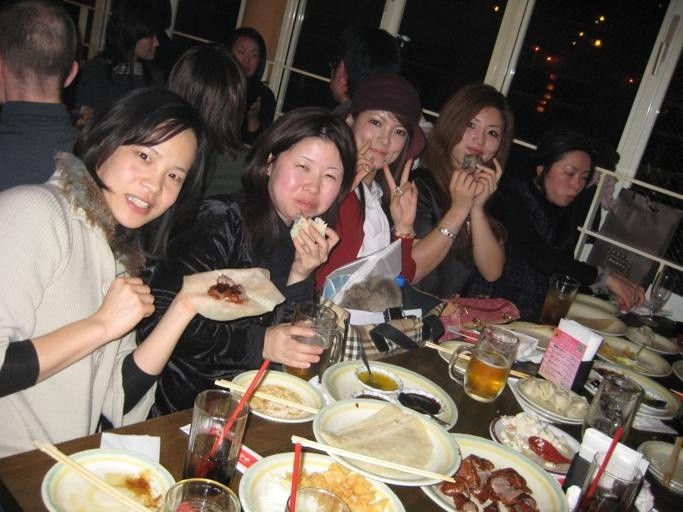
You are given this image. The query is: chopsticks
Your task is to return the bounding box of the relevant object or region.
[288,434,457,485]
[213,377,322,413]
[35,438,150,511]
[425,340,532,377]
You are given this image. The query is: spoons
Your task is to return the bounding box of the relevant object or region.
[354,327,381,390]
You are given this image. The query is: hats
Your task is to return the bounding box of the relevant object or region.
[339,74,427,158]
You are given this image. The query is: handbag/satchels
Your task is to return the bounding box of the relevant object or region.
[318,279,446,365]
[586,187,682,294]
[438,293,520,345]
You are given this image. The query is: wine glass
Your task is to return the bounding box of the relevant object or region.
[638,270,679,329]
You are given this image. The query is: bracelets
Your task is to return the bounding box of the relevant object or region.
[433,224,458,240]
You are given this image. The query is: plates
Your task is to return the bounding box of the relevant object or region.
[439,341,475,366]
[44,448,174,510]
[230,368,324,423]
[322,362,458,429]
[493,322,555,354]
[312,397,463,483]
[425,433,570,512]
[491,410,580,475]
[240,451,407,512]
[515,377,589,423]
[592,359,678,412]
[565,299,683,377]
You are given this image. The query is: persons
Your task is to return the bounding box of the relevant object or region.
[464,128,648,325]
[0,83,225,461]
[404,83,511,317]
[129,102,357,422]
[1,1,434,261]
[316,70,421,301]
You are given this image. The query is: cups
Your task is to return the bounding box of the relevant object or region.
[580,373,643,448]
[285,488,351,512]
[284,301,343,380]
[164,476,243,512]
[182,388,249,484]
[577,450,646,512]
[447,324,520,404]
